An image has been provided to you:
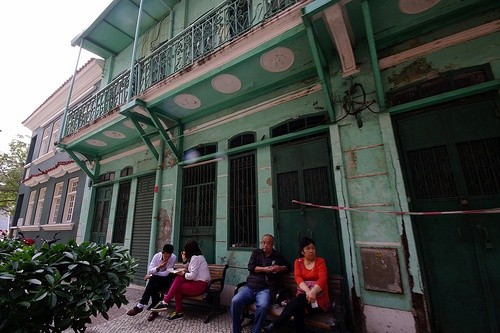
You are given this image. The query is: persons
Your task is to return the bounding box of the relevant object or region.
[230,234,291,333]
[150,240,212,321]
[126,243,176,321]
[263,236,330,333]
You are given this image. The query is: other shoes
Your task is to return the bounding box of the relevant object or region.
[147,311,158,321]
[263,322,276,333]
[128,306,142,316]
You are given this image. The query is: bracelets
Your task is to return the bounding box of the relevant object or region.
[150,274,154,279]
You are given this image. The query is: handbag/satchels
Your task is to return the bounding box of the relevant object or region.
[271,280,282,303]
[296,280,324,297]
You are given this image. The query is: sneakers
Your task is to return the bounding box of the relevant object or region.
[150,301,168,311]
[167,312,183,321]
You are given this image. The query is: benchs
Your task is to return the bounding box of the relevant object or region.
[234,271,346,333]
[159,263,228,324]
